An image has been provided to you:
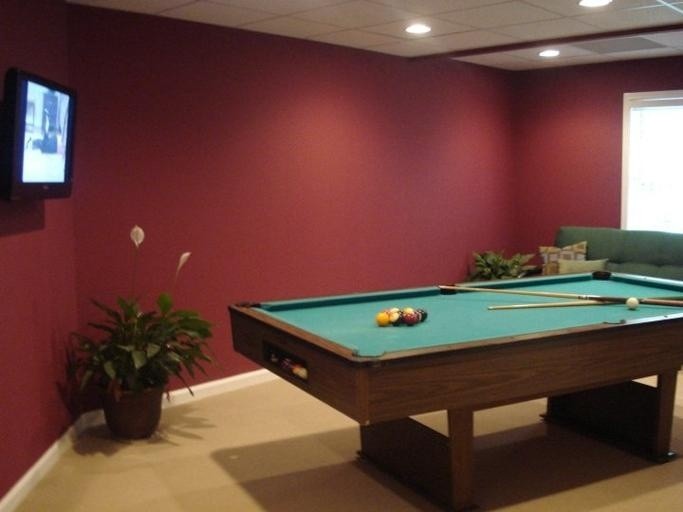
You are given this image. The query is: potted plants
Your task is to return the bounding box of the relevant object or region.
[97,375,169,439]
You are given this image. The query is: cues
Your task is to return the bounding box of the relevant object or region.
[439,285,683,306]
[489,296,683,310]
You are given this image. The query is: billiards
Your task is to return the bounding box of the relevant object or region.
[626,297,639,310]
[271,352,308,379]
[377,307,426,327]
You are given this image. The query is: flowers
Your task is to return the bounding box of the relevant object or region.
[58,225,213,401]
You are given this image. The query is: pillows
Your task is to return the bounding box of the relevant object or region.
[537,241,587,277]
[554,258,611,275]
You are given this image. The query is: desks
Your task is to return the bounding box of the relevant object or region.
[227,269,681,511]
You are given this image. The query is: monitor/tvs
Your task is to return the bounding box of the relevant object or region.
[10,76,74,202]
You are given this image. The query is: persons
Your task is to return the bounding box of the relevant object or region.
[41,106,49,147]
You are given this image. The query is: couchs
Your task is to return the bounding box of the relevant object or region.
[552,225,682,281]
